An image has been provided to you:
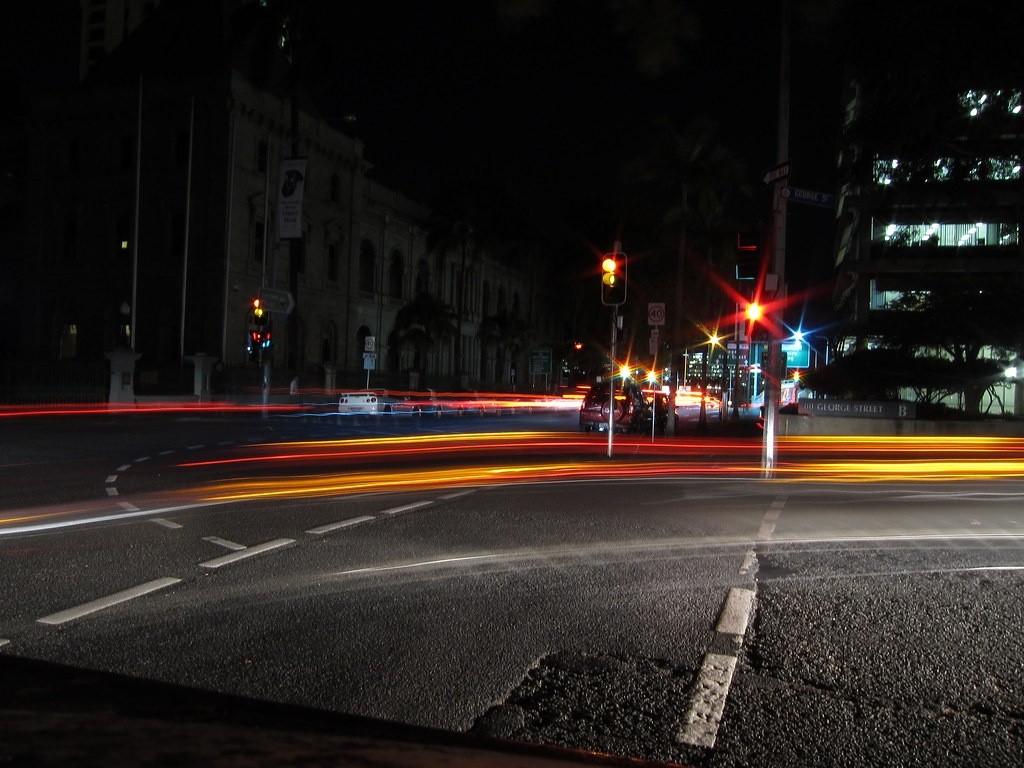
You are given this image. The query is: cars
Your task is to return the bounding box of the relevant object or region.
[642,390,680,432]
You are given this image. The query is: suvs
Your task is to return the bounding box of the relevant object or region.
[578,378,650,434]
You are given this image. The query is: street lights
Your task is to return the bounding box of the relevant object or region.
[260,109,357,419]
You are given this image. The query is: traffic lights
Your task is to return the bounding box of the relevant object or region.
[600,251,628,306]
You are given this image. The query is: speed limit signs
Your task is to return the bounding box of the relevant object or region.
[646,302,666,326]
